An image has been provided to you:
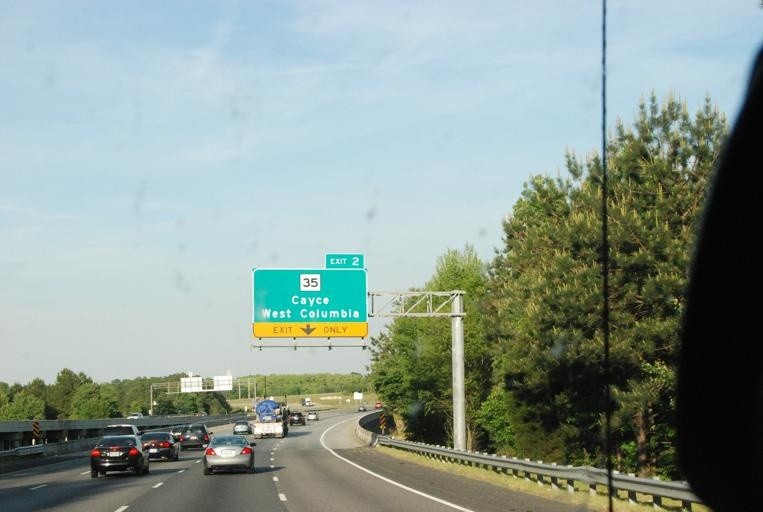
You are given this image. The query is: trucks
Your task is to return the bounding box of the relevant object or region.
[252,394,291,441]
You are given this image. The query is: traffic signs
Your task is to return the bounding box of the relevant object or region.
[253,268,367,323]
[325,252,366,269]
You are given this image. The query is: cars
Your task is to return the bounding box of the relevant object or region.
[126,412,146,422]
[358,405,367,411]
[194,411,208,418]
[89,423,257,478]
[233,394,320,434]
[375,401,381,409]
